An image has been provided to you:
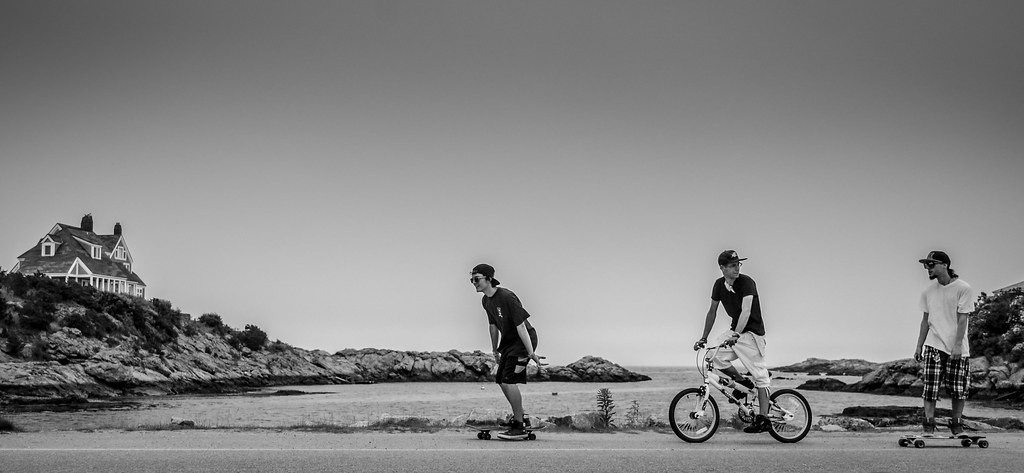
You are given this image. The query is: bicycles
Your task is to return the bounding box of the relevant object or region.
[668,338,813,443]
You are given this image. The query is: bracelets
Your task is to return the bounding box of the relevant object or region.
[493,353,498,356]
[528,353,535,359]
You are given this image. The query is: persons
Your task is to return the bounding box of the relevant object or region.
[912,250,975,437]
[469,264,548,440]
[693,249,772,433]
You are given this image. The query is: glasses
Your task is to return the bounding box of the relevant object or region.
[724,263,742,268]
[470,277,487,283]
[923,262,942,269]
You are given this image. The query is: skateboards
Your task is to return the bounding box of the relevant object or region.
[464,422,548,441]
[897,435,990,448]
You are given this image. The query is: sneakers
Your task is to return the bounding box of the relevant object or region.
[728,377,754,403]
[499,416,531,429]
[922,418,940,437]
[948,417,968,437]
[497,420,528,439]
[744,414,772,433]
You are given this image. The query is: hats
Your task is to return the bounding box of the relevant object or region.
[473,264,500,285]
[919,251,950,267]
[718,250,747,264]
